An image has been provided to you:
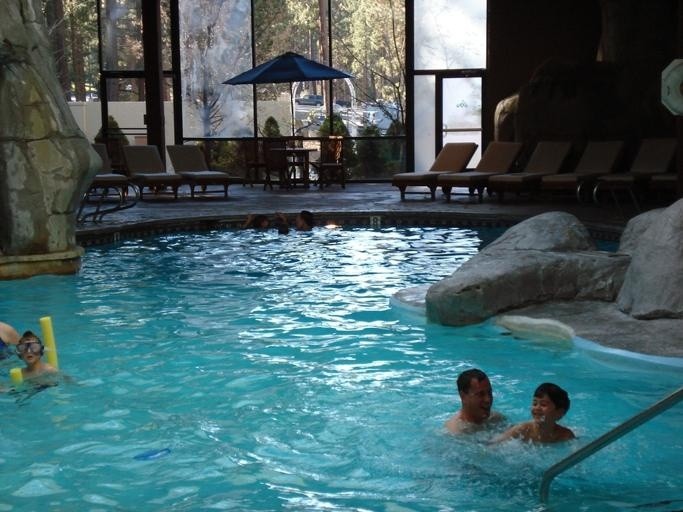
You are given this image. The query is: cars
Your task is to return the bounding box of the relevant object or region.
[89,92,98,102]
[293,93,323,108]
[361,99,384,110]
[332,98,350,109]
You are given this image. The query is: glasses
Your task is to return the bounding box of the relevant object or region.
[16,342,43,353]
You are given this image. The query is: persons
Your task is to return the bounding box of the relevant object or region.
[0,320,23,362]
[14,331,55,380]
[246,210,314,233]
[488,382,573,445]
[435,369,515,434]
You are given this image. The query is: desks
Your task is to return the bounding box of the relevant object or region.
[277,146,318,191]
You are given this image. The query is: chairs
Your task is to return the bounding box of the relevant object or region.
[121,143,185,199]
[392,139,479,200]
[542,137,630,201]
[316,134,348,190]
[238,137,276,187]
[166,143,231,199]
[261,135,297,192]
[590,137,682,212]
[487,138,572,201]
[85,142,131,204]
[438,139,526,203]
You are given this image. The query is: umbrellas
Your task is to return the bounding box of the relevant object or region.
[221,49,355,188]
